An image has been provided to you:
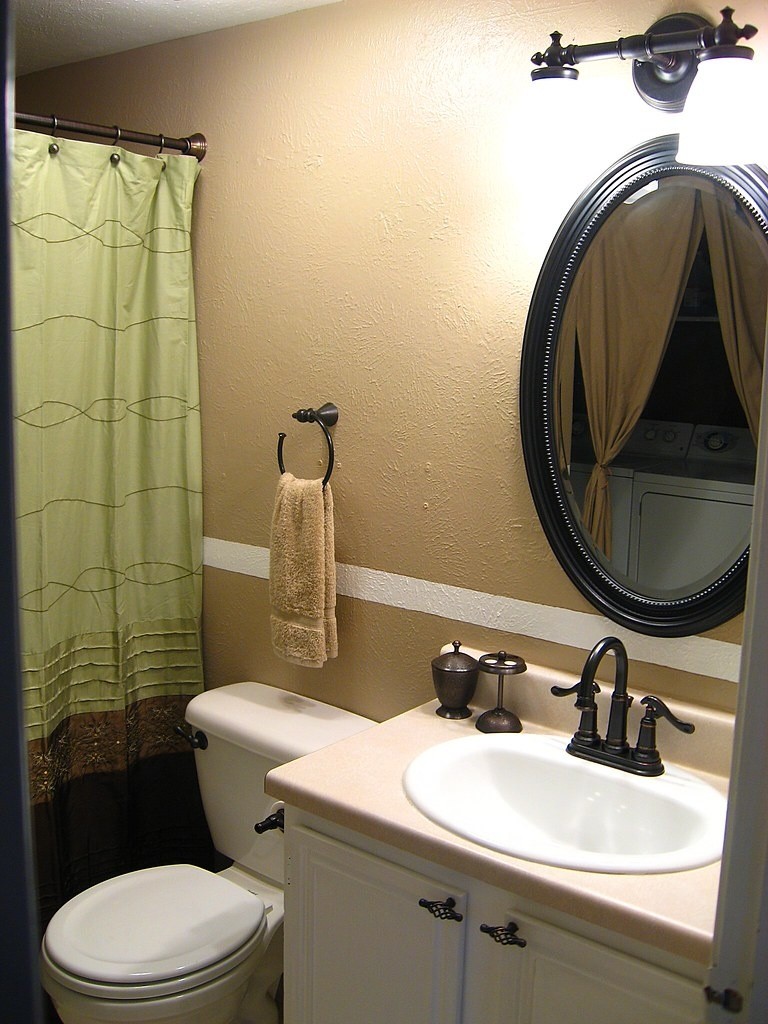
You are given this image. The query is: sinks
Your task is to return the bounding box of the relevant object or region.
[397,727,731,879]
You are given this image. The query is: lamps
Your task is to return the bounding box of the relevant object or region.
[529,8,768,167]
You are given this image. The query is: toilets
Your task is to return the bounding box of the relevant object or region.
[33,681,385,1023]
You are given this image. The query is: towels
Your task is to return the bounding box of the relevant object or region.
[264,469,342,671]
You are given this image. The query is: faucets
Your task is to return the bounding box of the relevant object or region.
[548,635,699,781]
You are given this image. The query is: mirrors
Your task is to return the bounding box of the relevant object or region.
[518,135,768,642]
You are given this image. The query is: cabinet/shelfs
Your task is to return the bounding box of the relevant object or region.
[284,803,708,1024]
